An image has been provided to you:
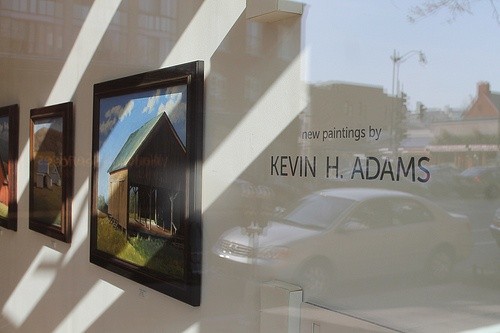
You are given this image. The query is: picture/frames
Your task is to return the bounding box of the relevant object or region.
[86,58,206,310]
[0,104,19,234]
[28,100,75,245]
